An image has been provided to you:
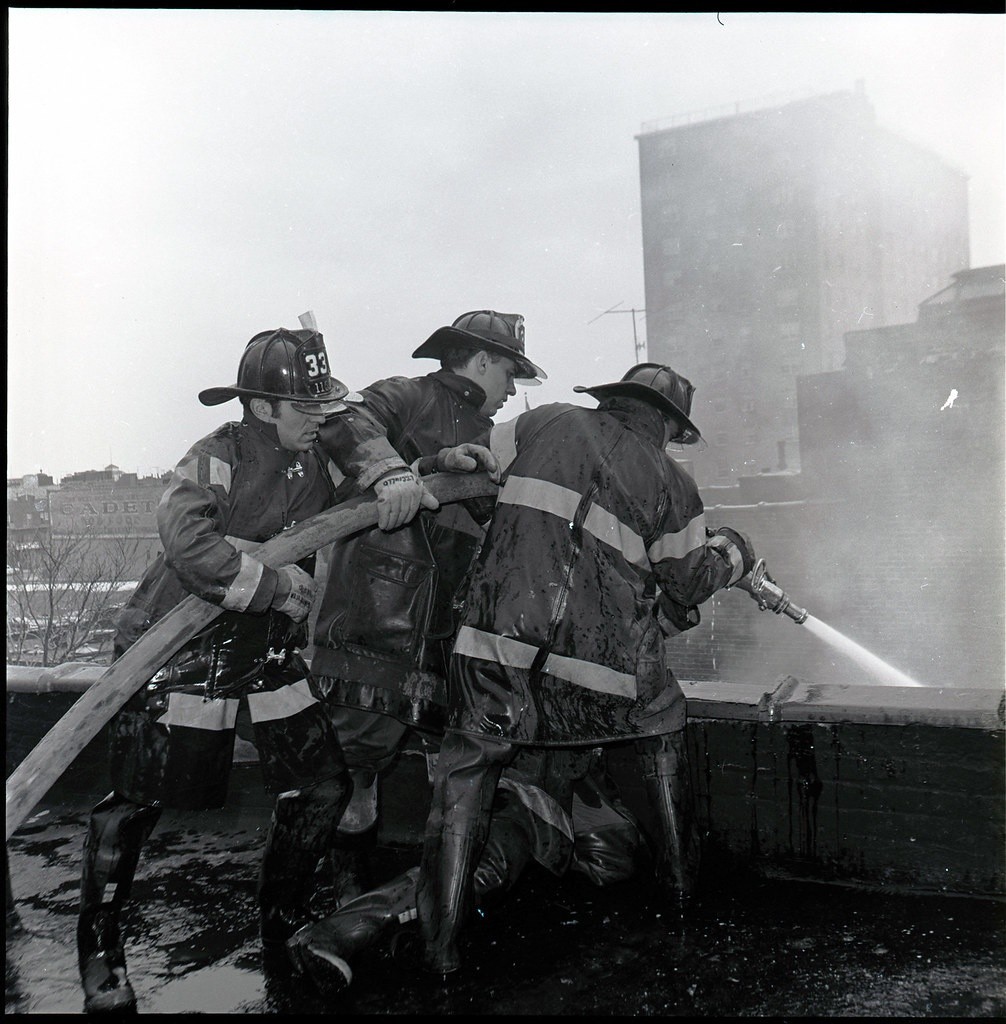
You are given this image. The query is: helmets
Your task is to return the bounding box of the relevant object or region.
[197,327,365,416]
[411,310,548,387]
[573,363,709,455]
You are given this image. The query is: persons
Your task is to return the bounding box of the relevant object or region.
[286,590,702,994]
[261,310,549,930]
[75,328,441,1012]
[408,361,763,978]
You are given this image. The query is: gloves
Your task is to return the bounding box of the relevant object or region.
[436,442,502,485]
[373,467,439,531]
[270,563,318,624]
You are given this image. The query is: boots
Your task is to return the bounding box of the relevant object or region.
[76,790,164,1014]
[645,779,696,908]
[257,778,355,951]
[283,816,634,997]
[415,833,483,1015]
